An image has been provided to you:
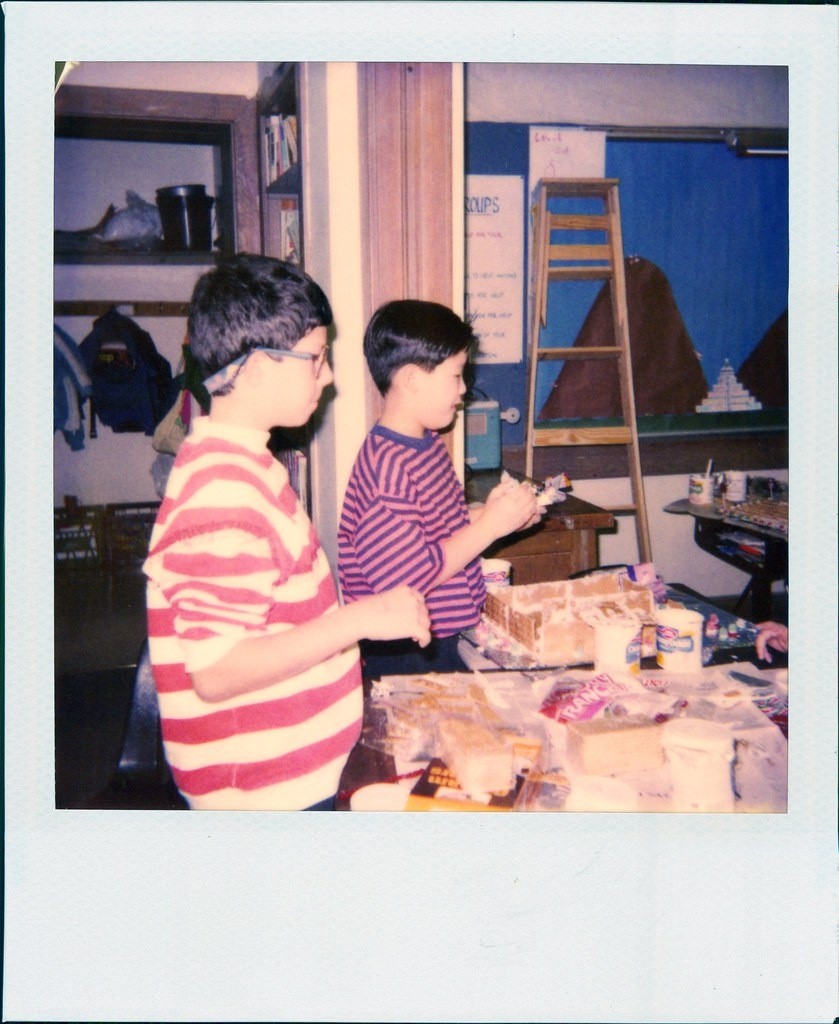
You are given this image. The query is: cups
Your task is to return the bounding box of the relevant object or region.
[655,609,705,669]
[594,622,642,671]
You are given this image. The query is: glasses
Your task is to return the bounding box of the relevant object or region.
[254,344,330,380]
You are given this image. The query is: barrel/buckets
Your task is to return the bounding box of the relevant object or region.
[156,195,215,251]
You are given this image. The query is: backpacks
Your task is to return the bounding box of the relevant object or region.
[77,304,173,439]
[54,322,89,451]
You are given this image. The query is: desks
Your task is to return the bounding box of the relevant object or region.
[467,467,615,584]
[663,498,789,624]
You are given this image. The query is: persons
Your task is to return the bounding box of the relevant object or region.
[755,621,788,663]
[337,299,567,682]
[138,252,433,811]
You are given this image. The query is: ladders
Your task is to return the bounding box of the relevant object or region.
[521,175,655,573]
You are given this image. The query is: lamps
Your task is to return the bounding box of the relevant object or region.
[727,129,788,157]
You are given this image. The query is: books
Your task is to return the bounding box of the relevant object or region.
[279,447,309,514]
[258,113,302,194]
[712,526,764,565]
[270,200,302,264]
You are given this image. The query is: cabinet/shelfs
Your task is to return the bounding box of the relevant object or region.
[254,62,311,275]
[54,83,262,262]
